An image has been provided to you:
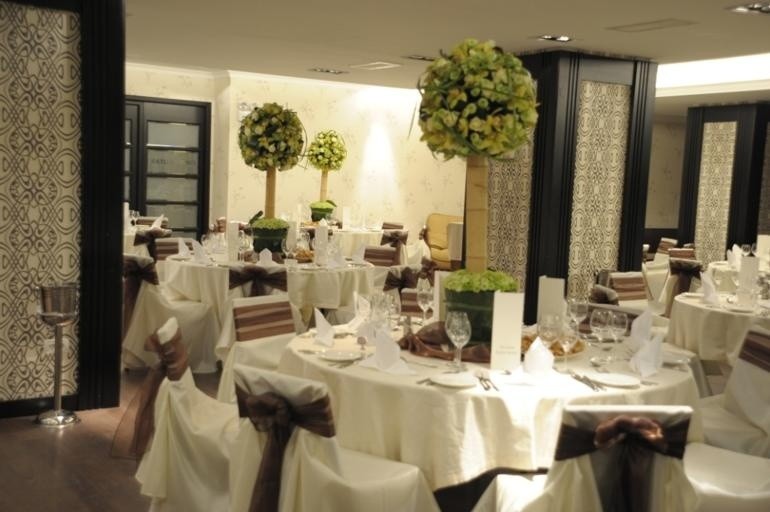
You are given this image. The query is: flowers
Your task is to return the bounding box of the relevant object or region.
[238,103,304,170]
[304,129,347,170]
[415,37,537,160]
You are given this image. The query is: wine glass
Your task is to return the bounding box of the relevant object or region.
[355,288,628,373]
[751,244,758,258]
[201,232,338,265]
[129,210,134,224]
[133,211,140,226]
[742,245,751,258]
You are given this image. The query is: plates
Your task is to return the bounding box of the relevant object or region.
[588,373,639,388]
[316,349,364,362]
[300,263,325,270]
[730,305,754,313]
[170,256,191,260]
[682,292,704,297]
[431,374,480,387]
[661,351,691,365]
